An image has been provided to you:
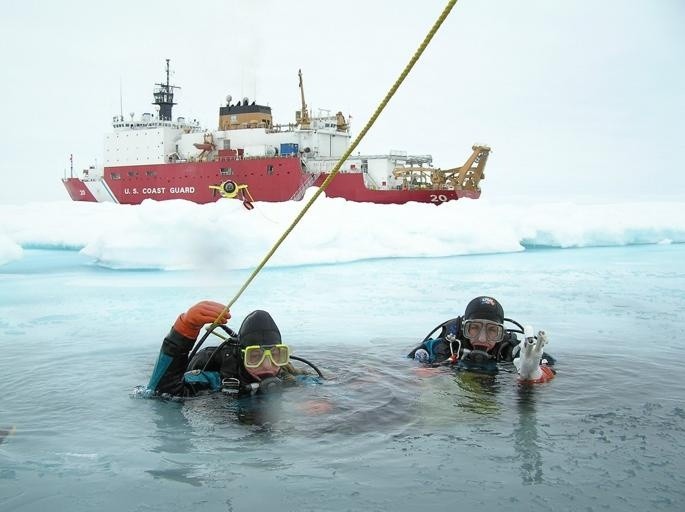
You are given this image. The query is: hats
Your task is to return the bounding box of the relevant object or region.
[463,295,503,354]
[236,310,282,384]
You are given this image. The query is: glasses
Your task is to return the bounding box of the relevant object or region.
[460,318,505,343]
[242,342,290,368]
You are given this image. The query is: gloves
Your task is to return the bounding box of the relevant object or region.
[171,300,230,340]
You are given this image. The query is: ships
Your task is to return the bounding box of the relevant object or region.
[57,53,492,205]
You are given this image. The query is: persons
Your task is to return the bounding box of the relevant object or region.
[407,296,555,385]
[148,301,322,400]
